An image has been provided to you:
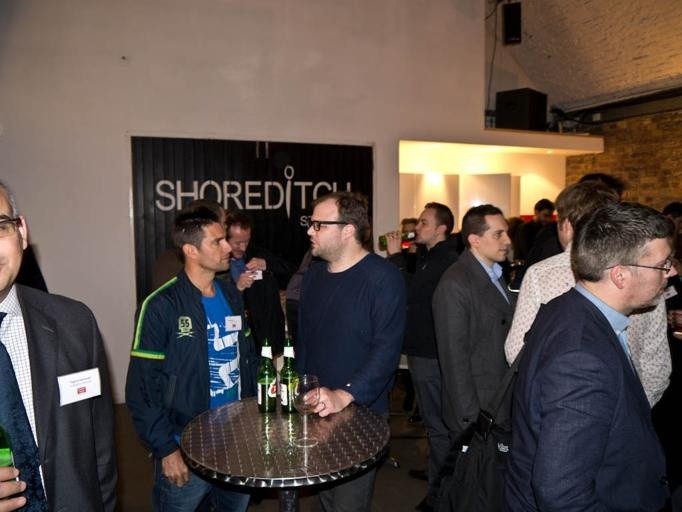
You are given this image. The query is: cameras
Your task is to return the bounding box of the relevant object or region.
[245,270,264,280]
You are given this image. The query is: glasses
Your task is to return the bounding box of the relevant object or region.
[309,220,348,231]
[605,258,673,275]
[0,217,21,238]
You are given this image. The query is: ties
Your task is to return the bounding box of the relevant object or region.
[0,312,48,511]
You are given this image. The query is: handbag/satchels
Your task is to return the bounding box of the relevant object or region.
[418,420,511,511]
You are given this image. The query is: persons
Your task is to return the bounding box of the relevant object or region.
[225,212,284,397]
[384,202,455,481]
[502,181,671,410]
[505,202,678,511]
[431,204,517,442]
[284,247,324,346]
[1,180,118,510]
[295,193,407,510]
[125,219,255,511]
[401,218,428,422]
[171,198,250,393]
[499,199,563,297]
[662,203,682,425]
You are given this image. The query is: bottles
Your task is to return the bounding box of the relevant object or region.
[379,231,418,248]
[0,424,30,512]
[280,339,299,413]
[257,336,276,412]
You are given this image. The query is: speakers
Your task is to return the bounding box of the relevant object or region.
[495,87,548,131]
[502,2,522,44]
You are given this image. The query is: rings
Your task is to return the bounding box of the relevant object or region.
[320,402,328,408]
[389,236,393,239]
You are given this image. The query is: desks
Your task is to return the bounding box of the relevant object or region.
[180,397,392,511]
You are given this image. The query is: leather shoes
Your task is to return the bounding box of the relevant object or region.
[408,467,429,480]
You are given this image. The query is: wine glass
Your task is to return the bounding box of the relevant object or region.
[291,374,320,448]
